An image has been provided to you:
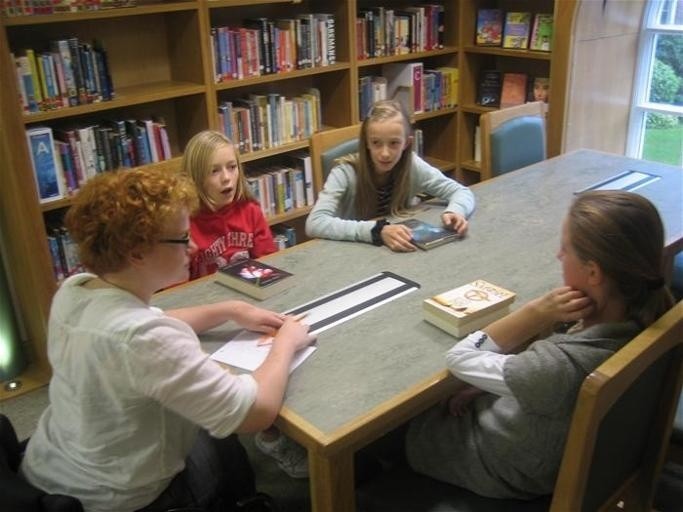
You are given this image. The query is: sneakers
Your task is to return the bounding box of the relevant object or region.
[255,432,309,479]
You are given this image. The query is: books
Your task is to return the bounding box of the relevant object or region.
[422,278,518,339]
[413,129,424,159]
[476,9,553,51]
[214,258,297,302]
[218,86,322,156]
[359,62,458,121]
[27,117,172,205]
[245,152,315,217]
[10,37,114,115]
[210,12,336,85]
[2,1,137,18]
[476,70,550,110]
[47,224,89,280]
[356,4,446,61]
[395,219,461,251]
[270,223,297,251]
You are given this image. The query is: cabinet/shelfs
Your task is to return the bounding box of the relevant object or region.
[203,0,459,226]
[456,0,646,189]
[0,0,215,376]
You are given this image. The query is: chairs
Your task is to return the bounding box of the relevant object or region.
[310,124,362,208]
[479,101,546,181]
[549,301,683,512]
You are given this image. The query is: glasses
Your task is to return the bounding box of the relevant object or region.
[158,232,193,247]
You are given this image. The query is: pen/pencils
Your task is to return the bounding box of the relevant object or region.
[259,313,309,342]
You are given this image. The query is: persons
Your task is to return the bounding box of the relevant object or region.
[405,189,676,500]
[163,129,309,479]
[16,164,319,512]
[305,99,476,254]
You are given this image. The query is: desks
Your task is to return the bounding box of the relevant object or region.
[150,148,682,512]
